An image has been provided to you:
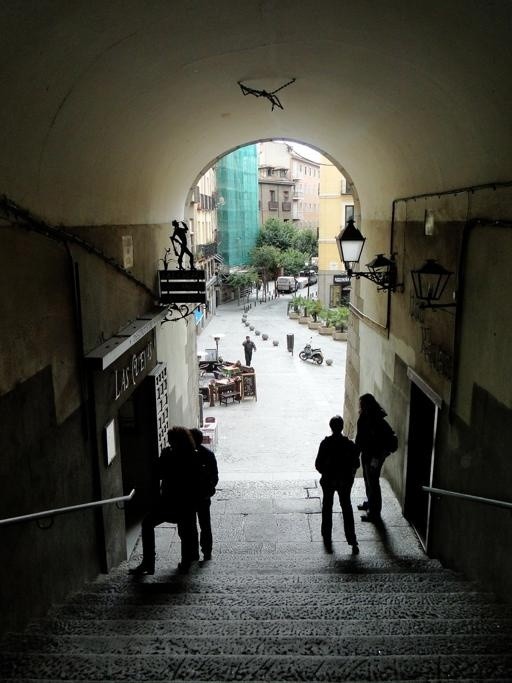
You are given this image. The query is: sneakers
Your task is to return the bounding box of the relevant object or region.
[348,539,358,546]
[357,500,370,520]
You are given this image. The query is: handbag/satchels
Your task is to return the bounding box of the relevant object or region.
[383,419,398,452]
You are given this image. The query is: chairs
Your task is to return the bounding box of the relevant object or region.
[200,416,219,453]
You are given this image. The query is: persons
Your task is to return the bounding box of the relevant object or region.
[355,392,393,522]
[127,423,201,575]
[242,335,256,366]
[315,415,362,556]
[187,427,220,561]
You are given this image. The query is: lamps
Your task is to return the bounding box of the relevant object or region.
[334,215,457,312]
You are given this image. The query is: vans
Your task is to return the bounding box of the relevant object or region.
[275,275,298,293]
[300,270,318,286]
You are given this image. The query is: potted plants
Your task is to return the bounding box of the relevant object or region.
[287,293,350,341]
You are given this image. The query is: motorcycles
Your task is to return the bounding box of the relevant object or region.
[299,337,323,365]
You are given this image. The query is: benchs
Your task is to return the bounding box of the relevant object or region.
[218,384,242,407]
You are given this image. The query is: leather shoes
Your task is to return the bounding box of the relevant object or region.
[128,563,155,575]
